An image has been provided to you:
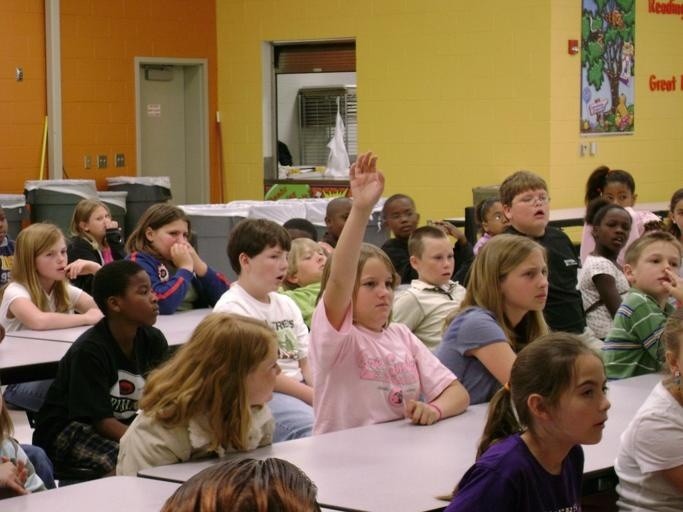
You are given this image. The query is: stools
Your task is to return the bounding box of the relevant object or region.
[52,467,102,480]
[5,402,39,428]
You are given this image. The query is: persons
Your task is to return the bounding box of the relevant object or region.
[162,458,320,512]
[577,165,682,381]
[115,312,282,475]
[0,200,231,332]
[214,169,585,440]
[32,260,169,485]
[615,302,682,511]
[310,151,470,436]
[0,408,55,501]
[446,333,612,512]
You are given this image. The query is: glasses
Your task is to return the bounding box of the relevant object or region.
[519,194,550,206]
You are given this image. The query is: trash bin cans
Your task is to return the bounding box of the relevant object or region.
[228,201,306,227]
[348,196,392,248]
[96,190,129,244]
[174,203,250,284]
[105,176,172,243]
[0,194,26,241]
[23,179,98,246]
[276,197,337,242]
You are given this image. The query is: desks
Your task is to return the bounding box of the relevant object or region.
[4,307,213,353]
[443,202,683,229]
[138,373,681,512]
[0,475,182,512]
[0,336,77,386]
